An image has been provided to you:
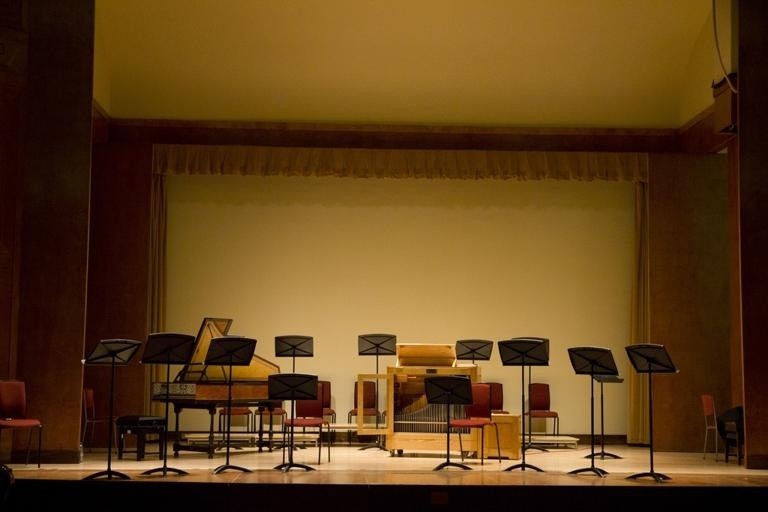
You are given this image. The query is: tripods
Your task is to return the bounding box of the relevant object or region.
[521,366,550,452]
[138,363,197,474]
[426,402,472,471]
[628,364,669,486]
[82,364,143,481]
[586,383,619,461]
[569,377,608,479]
[357,353,396,452]
[206,362,251,476]
[215,404,242,452]
[267,372,316,473]
[274,354,314,449]
[503,367,545,474]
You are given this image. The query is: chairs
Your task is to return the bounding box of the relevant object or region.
[447,384,502,465]
[524,383,560,437]
[283,384,330,465]
[348,381,381,448]
[0,381,43,468]
[254,400,287,444]
[82,388,119,454]
[479,382,509,414]
[304,381,337,444]
[219,407,253,444]
[721,418,745,466]
[702,394,734,462]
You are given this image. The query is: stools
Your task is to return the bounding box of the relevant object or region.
[116,414,166,461]
